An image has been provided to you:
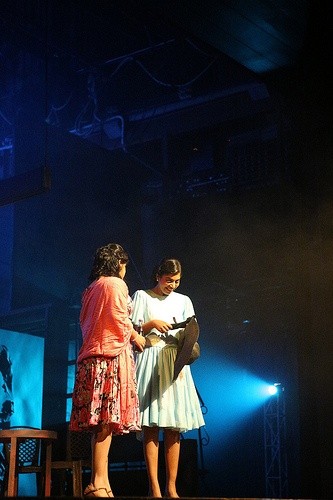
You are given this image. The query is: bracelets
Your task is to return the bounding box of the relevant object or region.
[131,330,138,342]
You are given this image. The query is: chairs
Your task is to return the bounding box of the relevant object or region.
[3,426,45,497]
[52,422,92,497]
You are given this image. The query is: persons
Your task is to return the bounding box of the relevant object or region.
[129,259,206,500]
[70,242,146,500]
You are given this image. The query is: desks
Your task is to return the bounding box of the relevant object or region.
[0,430,58,497]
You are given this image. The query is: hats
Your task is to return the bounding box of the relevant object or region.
[172,317,200,382]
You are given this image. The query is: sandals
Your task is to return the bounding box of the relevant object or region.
[84,485,113,500]
